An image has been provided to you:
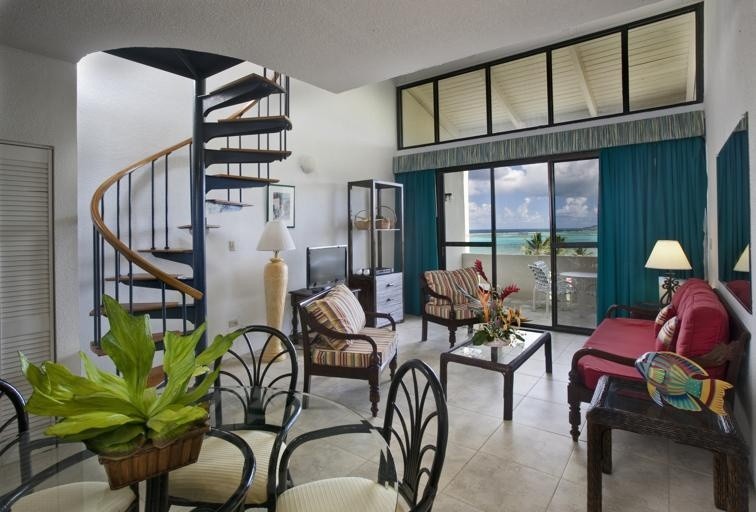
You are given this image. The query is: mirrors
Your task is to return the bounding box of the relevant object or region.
[716,112,753,316]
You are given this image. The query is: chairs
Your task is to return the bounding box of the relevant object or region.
[167,325,297,512]
[528,260,596,318]
[275,358,449,512]
[0,378,138,512]
[298,286,397,417]
[419,270,497,350]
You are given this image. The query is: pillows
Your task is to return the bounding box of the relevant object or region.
[425,266,480,305]
[306,284,366,349]
[656,315,679,351]
[654,304,675,338]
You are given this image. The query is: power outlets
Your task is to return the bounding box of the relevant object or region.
[227,320,239,328]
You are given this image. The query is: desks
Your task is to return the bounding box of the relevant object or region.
[289,288,361,344]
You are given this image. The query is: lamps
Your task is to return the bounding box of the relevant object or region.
[645,240,692,305]
[733,244,749,272]
[445,192,455,201]
[301,156,321,174]
[256,221,295,363]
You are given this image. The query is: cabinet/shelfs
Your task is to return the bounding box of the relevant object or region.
[348,178,404,328]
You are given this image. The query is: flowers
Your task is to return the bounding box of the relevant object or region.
[456,260,526,346]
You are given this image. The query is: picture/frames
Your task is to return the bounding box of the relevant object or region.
[267,184,296,229]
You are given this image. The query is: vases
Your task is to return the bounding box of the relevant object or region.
[479,323,512,347]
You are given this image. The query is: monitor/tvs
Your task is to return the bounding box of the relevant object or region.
[307,244,349,294]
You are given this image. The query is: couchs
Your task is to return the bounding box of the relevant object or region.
[727,279,751,310]
[567,278,751,442]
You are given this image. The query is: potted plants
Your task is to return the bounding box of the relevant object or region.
[19,295,253,490]
